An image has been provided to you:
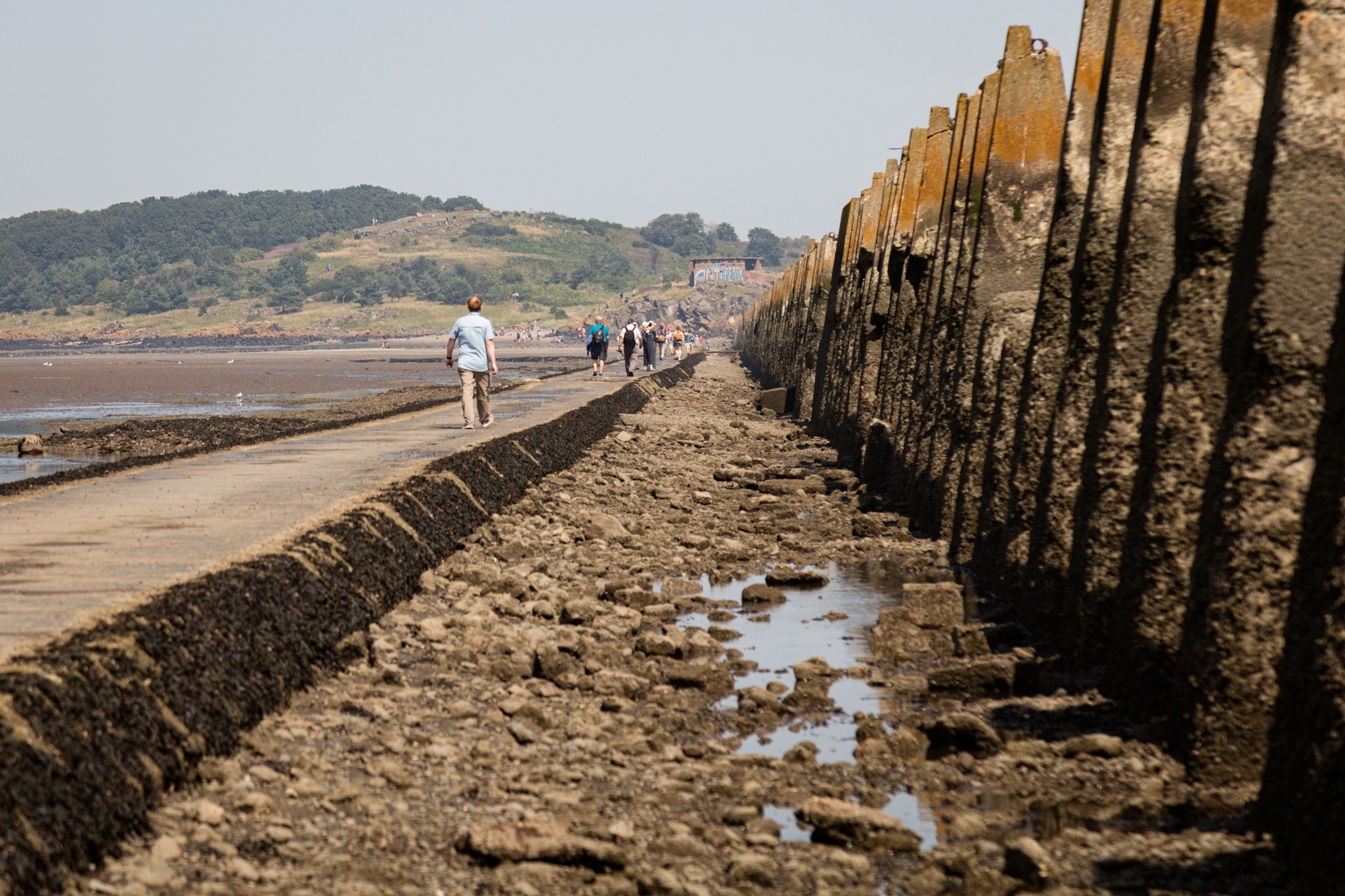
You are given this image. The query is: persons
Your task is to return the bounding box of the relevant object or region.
[577,320,586,337]
[552,324,555,332]
[658,325,703,361]
[372,218,380,226]
[493,325,505,335]
[512,292,519,302]
[618,319,657,376]
[705,339,709,351]
[578,304,582,310]
[381,342,391,348]
[516,318,542,343]
[606,288,635,308]
[447,296,498,429]
[432,212,456,224]
[587,315,609,375]
[659,275,663,283]
[326,264,331,273]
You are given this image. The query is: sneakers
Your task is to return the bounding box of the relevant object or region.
[462,424,473,429]
[482,414,493,427]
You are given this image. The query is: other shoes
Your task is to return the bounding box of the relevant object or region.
[674,357,678,360]
[659,358,663,360]
[627,374,630,376]
[599,373,602,375]
[643,367,648,370]
[593,372,597,376]
[649,367,656,370]
[629,372,633,376]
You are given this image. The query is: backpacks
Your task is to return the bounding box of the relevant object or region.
[623,327,636,346]
[675,332,682,341]
[642,329,648,342]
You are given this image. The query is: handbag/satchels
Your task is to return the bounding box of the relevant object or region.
[593,330,604,345]
[657,335,665,342]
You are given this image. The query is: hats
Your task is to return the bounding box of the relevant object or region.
[628,319,634,323]
[642,322,649,329]
[676,326,680,330]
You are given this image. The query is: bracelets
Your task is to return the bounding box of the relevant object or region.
[446,356,452,359]
